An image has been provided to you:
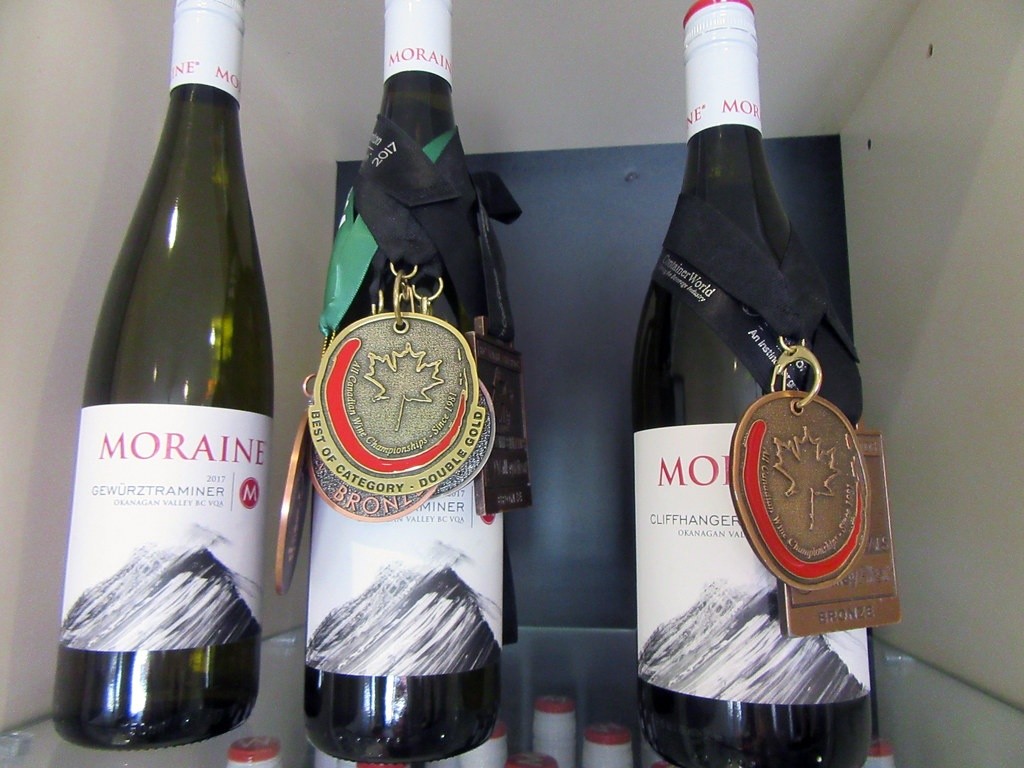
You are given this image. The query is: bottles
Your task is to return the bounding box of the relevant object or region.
[52,0,276,750]
[867,741,896,768]
[303,0,510,761]
[226,735,281,768]
[316,720,509,768]
[631,0,874,768]
[0,730,36,768]
[585,721,634,768]
[513,754,559,768]
[531,693,577,768]
